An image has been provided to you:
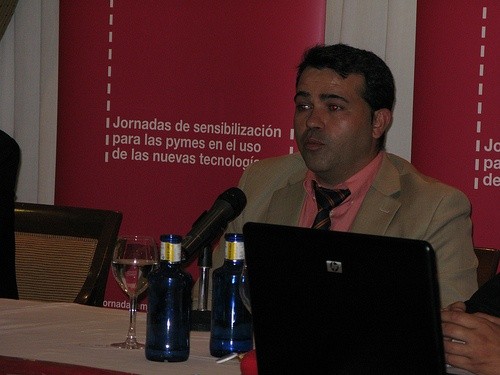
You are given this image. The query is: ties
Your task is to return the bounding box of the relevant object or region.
[310,180,351,229]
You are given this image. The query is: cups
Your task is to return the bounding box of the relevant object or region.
[189,266,214,330]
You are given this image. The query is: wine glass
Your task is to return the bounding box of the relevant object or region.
[110,235,158,350]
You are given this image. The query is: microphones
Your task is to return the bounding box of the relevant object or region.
[131,186,247,295]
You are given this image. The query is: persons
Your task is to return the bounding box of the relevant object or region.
[0,0,22,300]
[191,43,477,311]
[438,276,500,375]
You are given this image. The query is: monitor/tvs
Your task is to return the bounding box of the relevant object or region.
[242,221,450,375]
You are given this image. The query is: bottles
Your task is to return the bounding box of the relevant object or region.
[209,232,253,358]
[145,233,193,362]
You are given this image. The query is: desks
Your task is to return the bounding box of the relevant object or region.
[0,297,256,375]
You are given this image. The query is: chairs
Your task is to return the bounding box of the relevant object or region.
[13,202,123,307]
